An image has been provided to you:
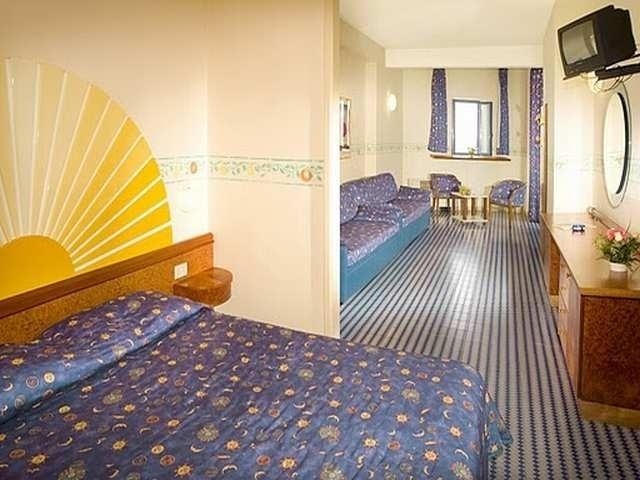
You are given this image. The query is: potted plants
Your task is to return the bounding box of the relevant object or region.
[466,189,471,195]
[459,186,466,196]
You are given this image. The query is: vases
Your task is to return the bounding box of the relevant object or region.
[608,263,628,282]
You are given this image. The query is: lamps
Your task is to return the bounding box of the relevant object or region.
[532,112,547,146]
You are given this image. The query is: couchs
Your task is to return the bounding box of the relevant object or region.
[340,173,431,304]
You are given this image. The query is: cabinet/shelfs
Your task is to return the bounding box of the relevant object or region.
[540,207,640,426]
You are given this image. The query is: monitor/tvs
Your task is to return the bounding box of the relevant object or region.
[556,4,638,80]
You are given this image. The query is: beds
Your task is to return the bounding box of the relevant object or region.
[0,232,513,480]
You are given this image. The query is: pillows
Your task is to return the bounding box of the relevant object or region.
[0,288,211,423]
[430,174,457,193]
[491,180,513,199]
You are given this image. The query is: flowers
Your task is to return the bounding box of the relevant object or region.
[592,226,639,263]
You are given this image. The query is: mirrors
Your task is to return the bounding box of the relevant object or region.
[602,80,632,209]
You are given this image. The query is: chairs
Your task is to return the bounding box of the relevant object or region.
[429,173,462,217]
[489,179,527,226]
